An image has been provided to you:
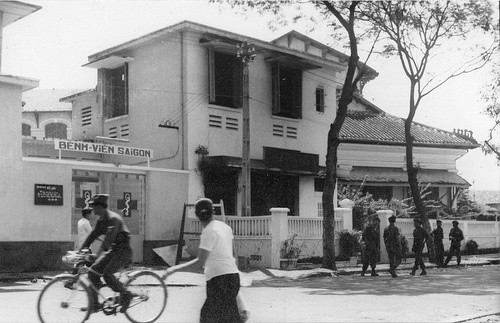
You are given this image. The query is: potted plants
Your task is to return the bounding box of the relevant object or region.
[280,234,307,270]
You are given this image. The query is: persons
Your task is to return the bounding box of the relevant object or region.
[430,220,464,268]
[64,208,107,289]
[80,194,132,313]
[361,218,380,276]
[410,218,426,275]
[161,199,240,323]
[384,216,402,277]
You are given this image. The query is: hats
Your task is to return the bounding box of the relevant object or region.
[452,221,459,224]
[372,218,380,222]
[388,216,396,220]
[88,193,110,206]
[414,218,422,223]
[437,220,442,223]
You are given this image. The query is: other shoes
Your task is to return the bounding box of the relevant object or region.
[438,264,447,268]
[420,271,426,275]
[361,270,365,276]
[390,270,397,277]
[410,271,415,275]
[456,264,459,267]
[371,270,378,276]
[120,293,133,312]
[82,304,100,311]
[64,283,75,289]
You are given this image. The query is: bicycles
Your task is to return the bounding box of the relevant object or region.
[37,251,168,322]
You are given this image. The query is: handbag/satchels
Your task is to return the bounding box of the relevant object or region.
[236,292,250,323]
